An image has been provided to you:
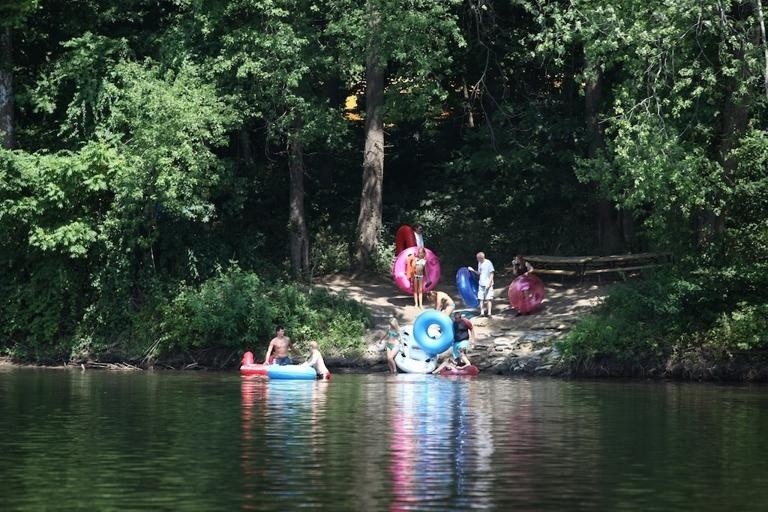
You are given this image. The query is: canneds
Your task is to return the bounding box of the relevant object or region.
[507,273,545,314]
[440,364,479,377]
[394,325,438,375]
[240,351,268,377]
[392,246,441,296]
[395,224,417,256]
[413,309,454,355]
[268,364,317,380]
[456,267,480,308]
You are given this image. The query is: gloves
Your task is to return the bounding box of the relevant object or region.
[238,351,318,380]
[392,324,439,376]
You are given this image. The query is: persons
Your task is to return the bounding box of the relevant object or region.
[414,248,430,309]
[512,254,534,316]
[468,251,495,316]
[430,348,472,374]
[451,312,475,344]
[303,340,330,380]
[263,325,295,366]
[429,289,455,314]
[413,223,425,247]
[379,317,404,373]
